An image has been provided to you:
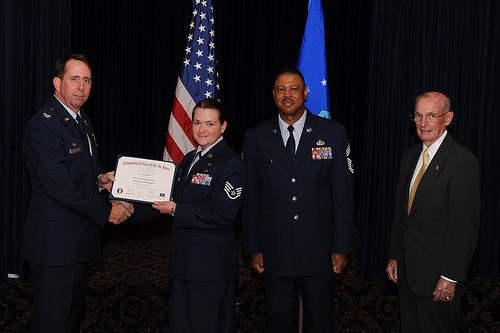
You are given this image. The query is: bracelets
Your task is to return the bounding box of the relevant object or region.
[171,203,176,216]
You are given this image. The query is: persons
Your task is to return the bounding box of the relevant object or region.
[385,91,481,333]
[241,70,354,332]
[20,53,131,333]
[109,99,246,333]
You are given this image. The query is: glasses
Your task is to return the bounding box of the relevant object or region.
[410,111,449,122]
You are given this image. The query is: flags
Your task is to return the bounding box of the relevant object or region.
[160,0,220,166]
[298,0,331,119]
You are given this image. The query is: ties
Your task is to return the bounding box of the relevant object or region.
[76,115,91,152]
[408,149,429,216]
[286,125,296,163]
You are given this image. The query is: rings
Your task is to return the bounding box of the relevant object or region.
[446,296,449,299]
